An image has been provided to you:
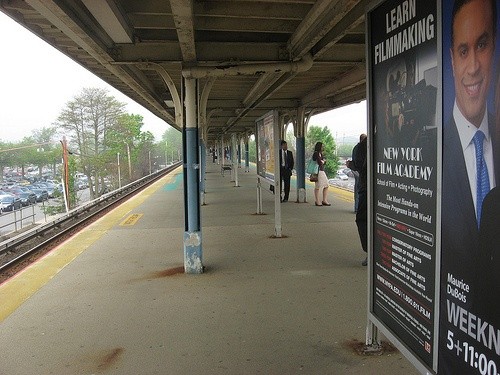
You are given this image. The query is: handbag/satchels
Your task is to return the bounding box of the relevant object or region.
[306,151,319,174]
[310,174,318,182]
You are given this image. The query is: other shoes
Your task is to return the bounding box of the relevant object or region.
[315,201,323,206]
[322,200,331,206]
[282,197,288,202]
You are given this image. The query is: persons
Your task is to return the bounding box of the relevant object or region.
[279,140,294,202]
[346,136,371,266]
[211,147,238,163]
[313,142,331,206]
[352,134,368,214]
[442,0,500,263]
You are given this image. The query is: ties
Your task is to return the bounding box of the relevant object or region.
[473,130,490,232]
[283,150,287,167]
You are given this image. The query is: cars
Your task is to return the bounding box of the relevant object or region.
[335,168,354,180]
[0,166,108,215]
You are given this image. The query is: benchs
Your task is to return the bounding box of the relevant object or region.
[221,164,232,178]
